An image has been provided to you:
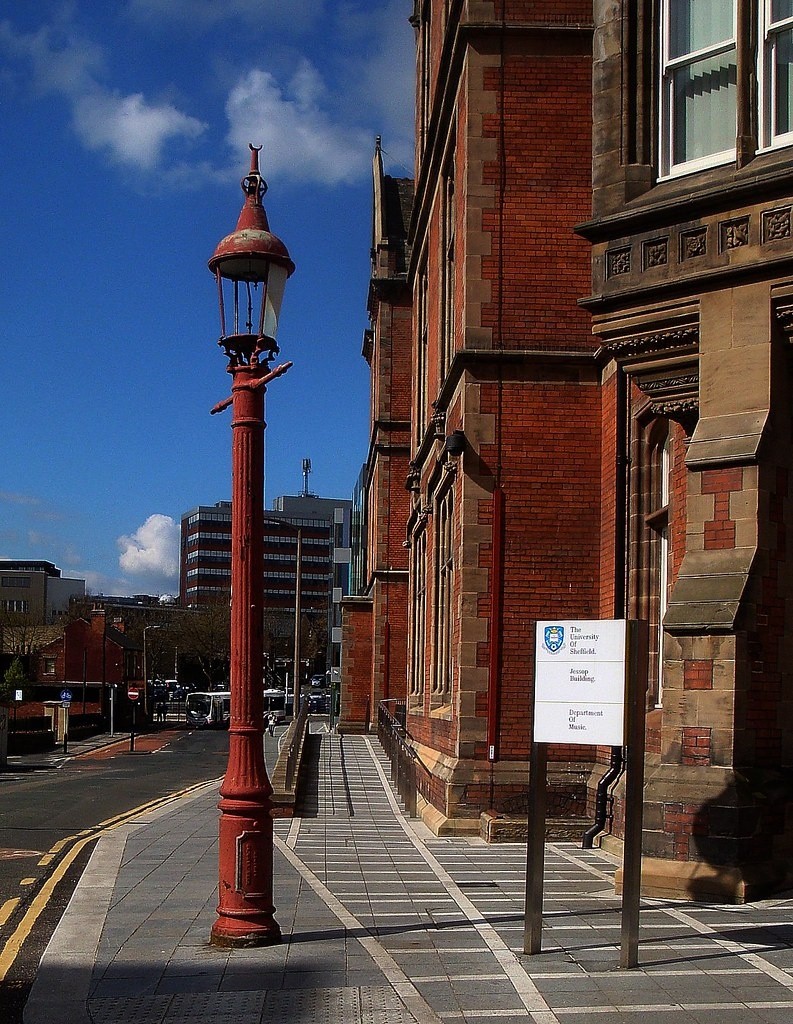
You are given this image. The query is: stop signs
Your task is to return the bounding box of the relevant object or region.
[128,687,139,699]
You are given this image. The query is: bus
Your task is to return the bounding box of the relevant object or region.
[186,687,287,729]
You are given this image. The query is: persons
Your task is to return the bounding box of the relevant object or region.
[162,702,168,721]
[156,703,162,723]
[263,711,270,728]
[268,712,277,737]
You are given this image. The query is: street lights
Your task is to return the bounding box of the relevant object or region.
[206,135,285,943]
[142,623,159,718]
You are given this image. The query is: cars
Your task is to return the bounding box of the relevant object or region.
[148,680,335,715]
